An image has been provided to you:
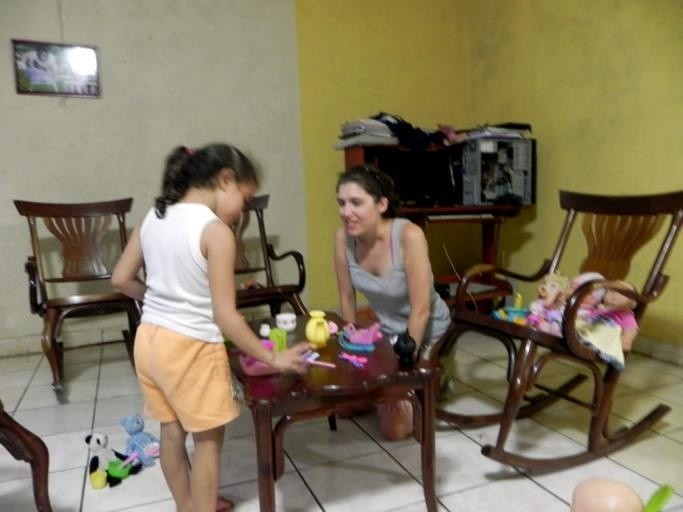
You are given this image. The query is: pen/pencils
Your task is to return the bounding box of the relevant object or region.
[307,359,335,369]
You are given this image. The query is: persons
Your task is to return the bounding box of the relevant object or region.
[111,139,317,512]
[333,164,453,442]
[494,266,640,373]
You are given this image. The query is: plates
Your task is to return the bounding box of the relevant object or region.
[143,442,161,457]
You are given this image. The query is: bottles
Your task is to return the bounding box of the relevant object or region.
[314,319,327,349]
[304,310,330,343]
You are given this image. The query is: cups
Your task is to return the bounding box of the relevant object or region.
[275,313,296,332]
[269,329,287,352]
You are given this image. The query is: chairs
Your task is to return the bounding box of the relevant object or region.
[230,193,308,320]
[431,185,682,473]
[12,196,143,393]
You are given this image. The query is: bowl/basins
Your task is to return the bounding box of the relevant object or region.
[106,461,132,478]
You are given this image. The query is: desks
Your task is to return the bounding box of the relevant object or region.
[392,202,512,319]
[224,310,447,511]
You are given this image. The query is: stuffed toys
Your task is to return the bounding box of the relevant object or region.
[84,431,143,488]
[118,412,161,466]
